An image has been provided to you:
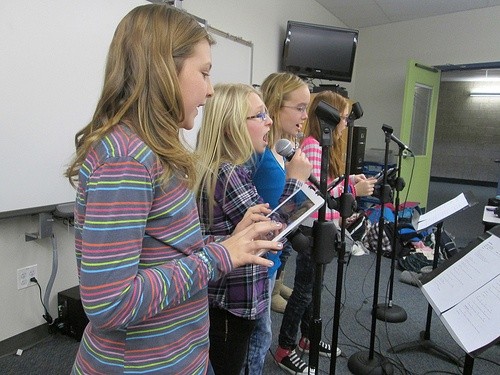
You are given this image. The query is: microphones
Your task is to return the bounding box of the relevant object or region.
[275,138,319,188]
[390,133,412,152]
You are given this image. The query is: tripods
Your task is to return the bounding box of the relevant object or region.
[388,190,479,368]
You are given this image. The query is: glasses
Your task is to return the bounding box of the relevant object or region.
[281,104,310,113]
[246,108,269,121]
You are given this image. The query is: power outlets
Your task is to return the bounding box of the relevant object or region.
[17,264,38,291]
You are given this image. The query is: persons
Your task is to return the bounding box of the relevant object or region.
[70,4,285,375]
[243,73,310,375]
[271,90,377,375]
[194,84,312,375]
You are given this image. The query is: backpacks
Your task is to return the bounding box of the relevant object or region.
[345,201,459,275]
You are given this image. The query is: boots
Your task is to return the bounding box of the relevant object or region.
[280,270,293,299]
[270,279,288,313]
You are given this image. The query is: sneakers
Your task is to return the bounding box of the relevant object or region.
[298,336,342,358]
[274,346,315,375]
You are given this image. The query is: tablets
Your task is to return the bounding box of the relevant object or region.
[251,184,327,258]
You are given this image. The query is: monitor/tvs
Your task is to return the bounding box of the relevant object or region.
[282,21,359,82]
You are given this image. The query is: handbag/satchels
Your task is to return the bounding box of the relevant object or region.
[209,308,264,375]
